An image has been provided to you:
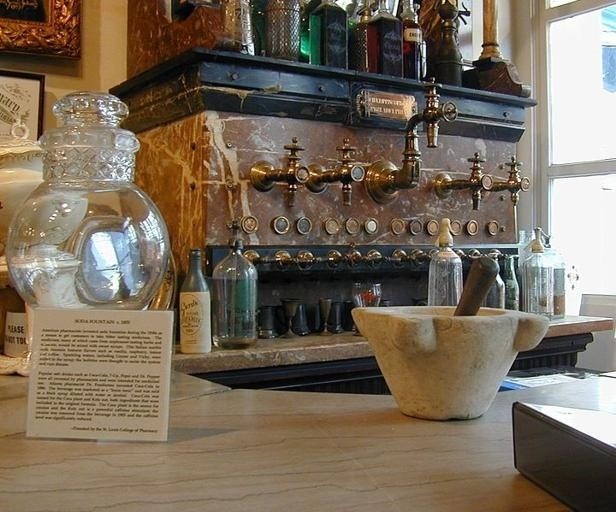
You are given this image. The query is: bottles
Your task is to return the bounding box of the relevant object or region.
[5,91,173,308]
[179,249,212,354]
[213,238,258,348]
[255,0,427,81]
[428,218,566,320]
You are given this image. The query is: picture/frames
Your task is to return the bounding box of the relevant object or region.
[0,0,81,61]
[0,70,45,142]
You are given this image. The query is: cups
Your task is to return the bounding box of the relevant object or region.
[258,281,391,339]
